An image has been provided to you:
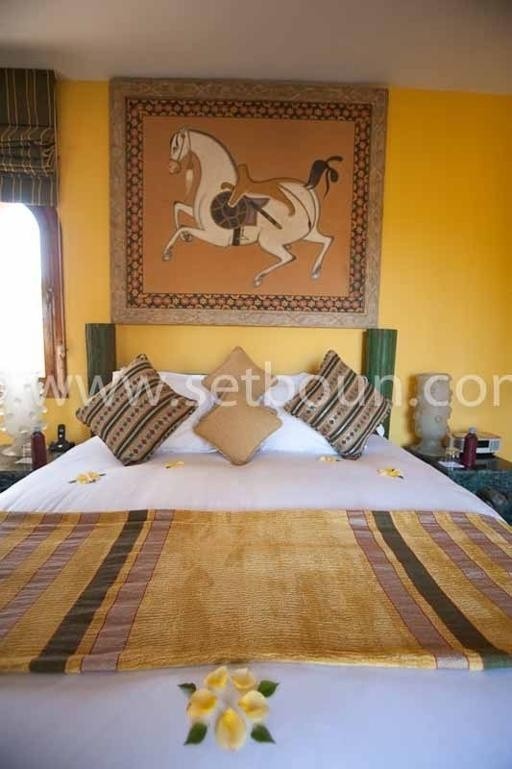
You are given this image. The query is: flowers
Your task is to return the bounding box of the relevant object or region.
[316,454,341,464]
[171,661,282,752]
[375,464,406,480]
[161,460,186,469]
[65,468,106,486]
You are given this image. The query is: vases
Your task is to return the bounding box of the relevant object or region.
[0,340,49,457]
[407,369,452,457]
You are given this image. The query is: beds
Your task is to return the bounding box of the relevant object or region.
[0,322,512,769]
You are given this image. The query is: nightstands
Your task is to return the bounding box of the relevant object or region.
[0,441,77,495]
[400,445,512,526]
[74,353,200,469]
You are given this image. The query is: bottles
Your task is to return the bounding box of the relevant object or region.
[464,427,478,468]
[32,426,47,471]
[51,424,71,460]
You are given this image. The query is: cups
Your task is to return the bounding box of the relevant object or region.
[444,448,461,466]
[16,439,32,472]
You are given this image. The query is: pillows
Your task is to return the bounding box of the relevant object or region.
[192,388,285,464]
[201,343,279,403]
[282,347,393,461]
[110,366,222,455]
[254,370,338,456]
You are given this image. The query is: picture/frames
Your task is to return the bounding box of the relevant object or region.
[107,75,389,331]
